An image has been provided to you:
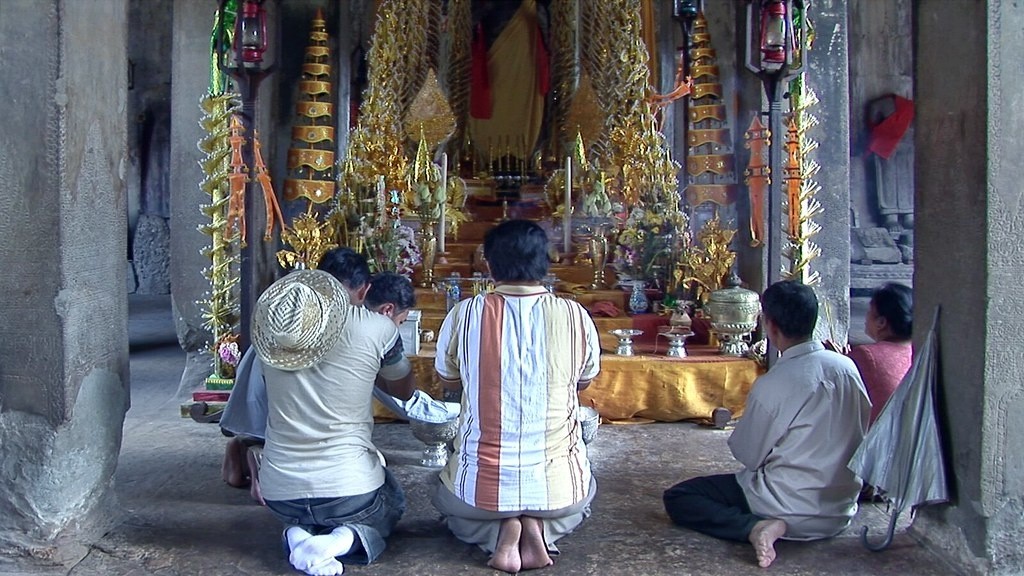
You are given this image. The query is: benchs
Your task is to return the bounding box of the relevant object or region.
[369,200,757,421]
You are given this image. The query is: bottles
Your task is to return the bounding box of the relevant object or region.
[446,281,461,313]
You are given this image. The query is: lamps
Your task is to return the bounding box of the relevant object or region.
[761,0,797,63]
[231,0,270,64]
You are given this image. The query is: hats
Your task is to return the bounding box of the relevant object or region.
[250,270,350,371]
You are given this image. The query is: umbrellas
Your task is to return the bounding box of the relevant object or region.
[846,305,951,552]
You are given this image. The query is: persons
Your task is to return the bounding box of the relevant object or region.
[219,247,415,576]
[820,283,914,429]
[432,218,602,573]
[662,278,914,568]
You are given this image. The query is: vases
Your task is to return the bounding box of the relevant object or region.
[586,219,612,287]
[670,308,694,329]
[629,280,647,315]
[215,353,238,380]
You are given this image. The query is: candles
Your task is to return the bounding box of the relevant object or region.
[562,157,571,253]
[437,152,449,251]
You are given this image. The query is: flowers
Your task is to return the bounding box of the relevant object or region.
[612,202,671,280]
[356,202,426,277]
[663,290,698,317]
[579,164,623,224]
[198,335,242,367]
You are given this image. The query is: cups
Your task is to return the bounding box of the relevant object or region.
[421,328,434,342]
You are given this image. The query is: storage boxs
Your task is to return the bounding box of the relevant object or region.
[399,309,424,356]
